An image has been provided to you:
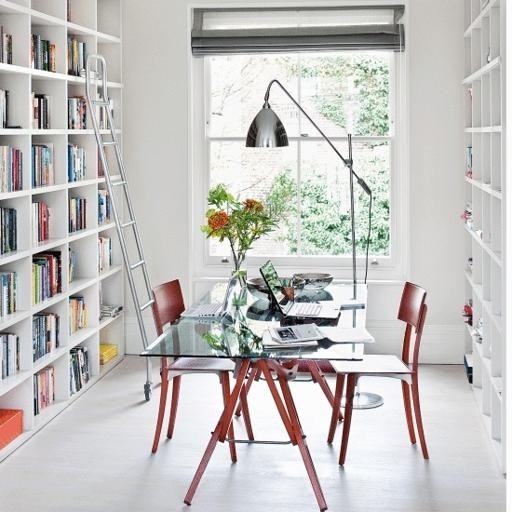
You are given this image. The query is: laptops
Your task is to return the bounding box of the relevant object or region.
[259,259,342,320]
[180,271,240,318]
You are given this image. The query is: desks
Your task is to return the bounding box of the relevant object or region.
[142,279,371,512]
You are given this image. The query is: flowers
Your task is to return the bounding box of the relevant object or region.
[203,179,280,274]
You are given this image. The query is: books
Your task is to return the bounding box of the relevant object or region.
[67,0,72,21]
[1,26,123,419]
[262,330,319,349]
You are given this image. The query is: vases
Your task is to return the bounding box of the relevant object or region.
[222,266,247,315]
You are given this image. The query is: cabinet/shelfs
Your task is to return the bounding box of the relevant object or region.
[458,0,508,479]
[0,0,124,465]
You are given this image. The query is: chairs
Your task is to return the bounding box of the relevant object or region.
[326,280,434,471]
[146,278,256,464]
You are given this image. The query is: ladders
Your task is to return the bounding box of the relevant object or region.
[85,54,168,402]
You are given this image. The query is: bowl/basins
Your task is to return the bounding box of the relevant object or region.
[244,276,293,300]
[292,272,334,289]
[295,290,334,302]
[245,300,280,322]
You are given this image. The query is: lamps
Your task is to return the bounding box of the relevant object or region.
[243,76,386,411]
